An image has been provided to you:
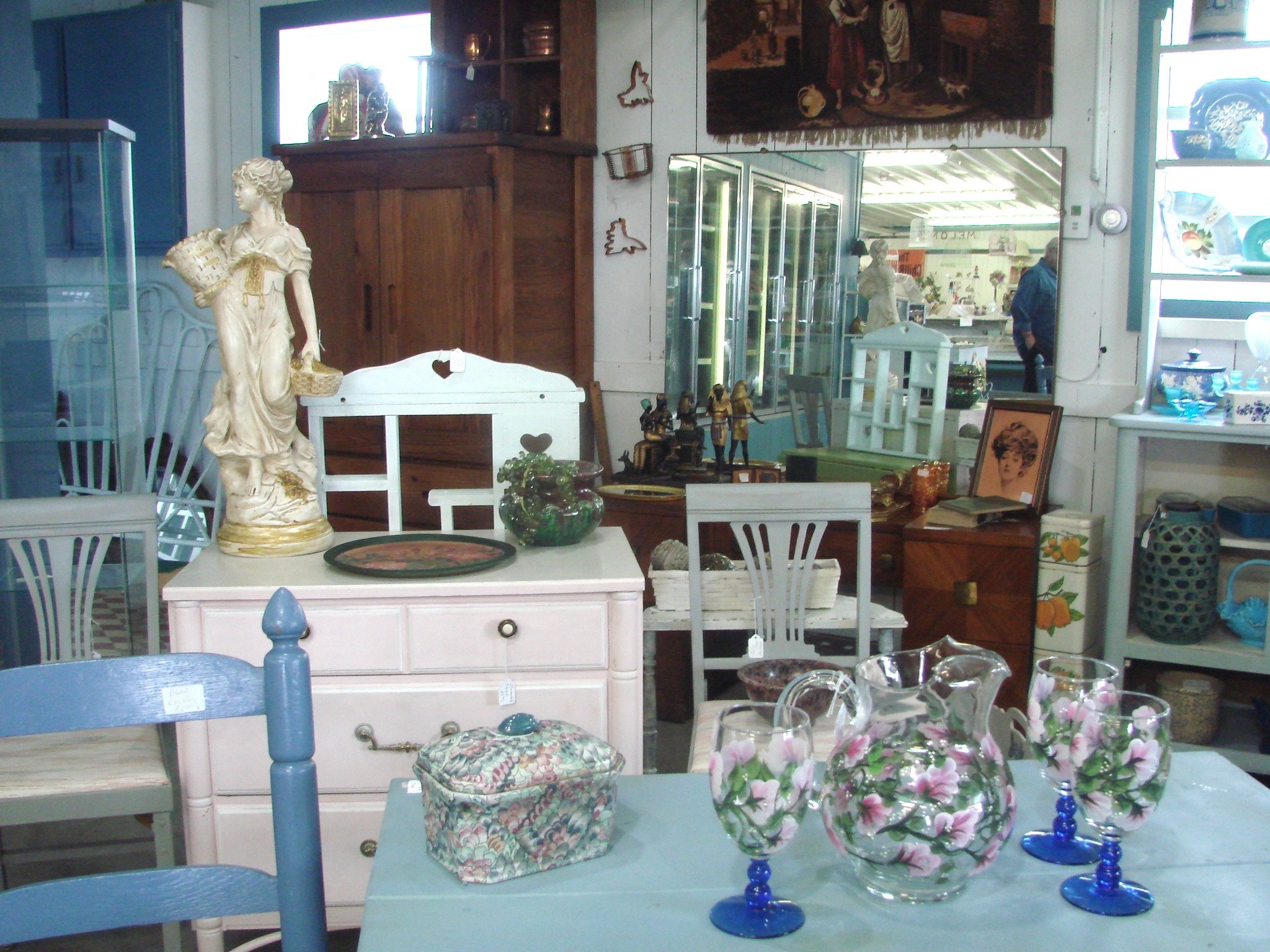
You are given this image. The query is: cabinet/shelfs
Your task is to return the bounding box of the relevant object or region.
[597,492,904,721]
[269,131,571,530]
[1106,0,1269,776]
[430,0,598,144]
[903,504,1063,736]
[664,154,841,416]
[162,532,645,952]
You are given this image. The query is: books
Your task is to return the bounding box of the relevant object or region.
[925,494,1027,528]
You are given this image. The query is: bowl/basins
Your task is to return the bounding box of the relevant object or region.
[1155,348,1230,402]
[738,656,850,726]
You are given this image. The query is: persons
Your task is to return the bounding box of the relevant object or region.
[1010,237,1058,394]
[859,240,901,360]
[640,380,765,471]
[194,156,322,495]
[366,83,395,139]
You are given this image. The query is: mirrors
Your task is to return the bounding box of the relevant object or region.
[662,148,1067,485]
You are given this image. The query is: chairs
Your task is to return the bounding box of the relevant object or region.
[685,482,872,771]
[1,491,182,952]
[0,587,331,952]
[787,375,834,448]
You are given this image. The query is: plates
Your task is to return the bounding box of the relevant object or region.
[1156,193,1269,275]
[1150,402,1216,417]
[593,484,687,501]
[323,532,515,575]
[1191,78,1270,159]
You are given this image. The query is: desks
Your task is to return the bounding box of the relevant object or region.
[642,592,911,773]
[360,752,1269,952]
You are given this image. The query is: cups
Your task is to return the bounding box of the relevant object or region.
[1170,129,1210,159]
[1156,671,1224,745]
[1189,0,1249,44]
[911,464,937,514]
[923,460,950,497]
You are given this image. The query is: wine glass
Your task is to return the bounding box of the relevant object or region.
[1020,653,1121,867]
[1059,690,1173,916]
[708,700,816,939]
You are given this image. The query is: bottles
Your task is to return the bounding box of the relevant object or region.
[1235,120,1268,160]
[537,104,559,136]
[1138,493,1217,645]
[522,18,559,56]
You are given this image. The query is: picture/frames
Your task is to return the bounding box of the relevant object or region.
[968,398,1064,517]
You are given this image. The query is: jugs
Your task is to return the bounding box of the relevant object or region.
[772,634,1019,906]
[464,28,494,61]
